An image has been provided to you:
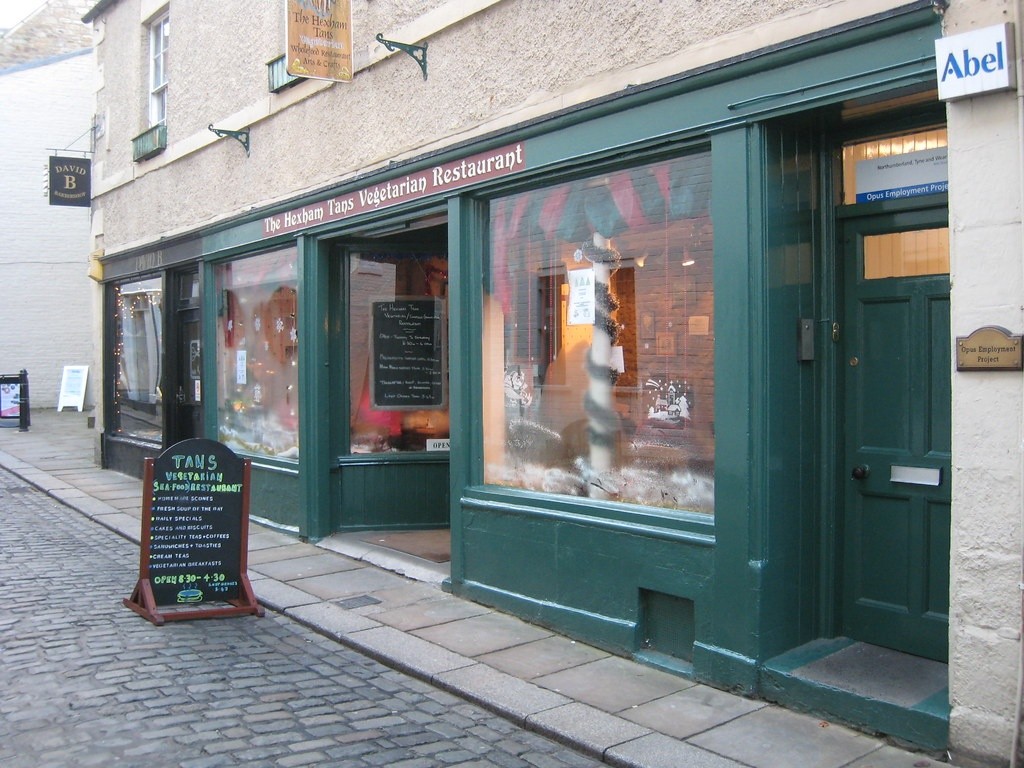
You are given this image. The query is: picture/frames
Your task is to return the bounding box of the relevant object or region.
[655,331,678,358]
[639,309,656,339]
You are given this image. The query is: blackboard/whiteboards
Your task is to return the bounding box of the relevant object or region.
[368,294,448,412]
[140,438,251,607]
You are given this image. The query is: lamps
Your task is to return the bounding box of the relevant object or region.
[681,242,695,267]
[634,248,649,269]
